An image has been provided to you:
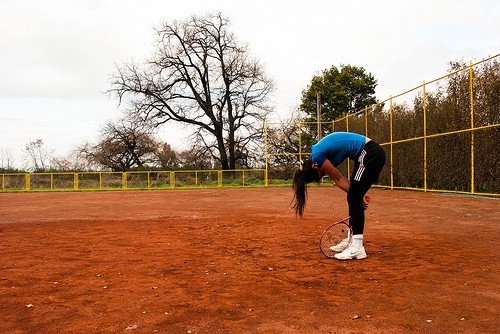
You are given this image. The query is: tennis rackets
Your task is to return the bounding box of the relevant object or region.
[319,195,371,259]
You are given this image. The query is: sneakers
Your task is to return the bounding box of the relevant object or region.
[334,246,367,259]
[329,238,352,252]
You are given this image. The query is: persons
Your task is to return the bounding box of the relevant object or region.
[288,132,385,260]
